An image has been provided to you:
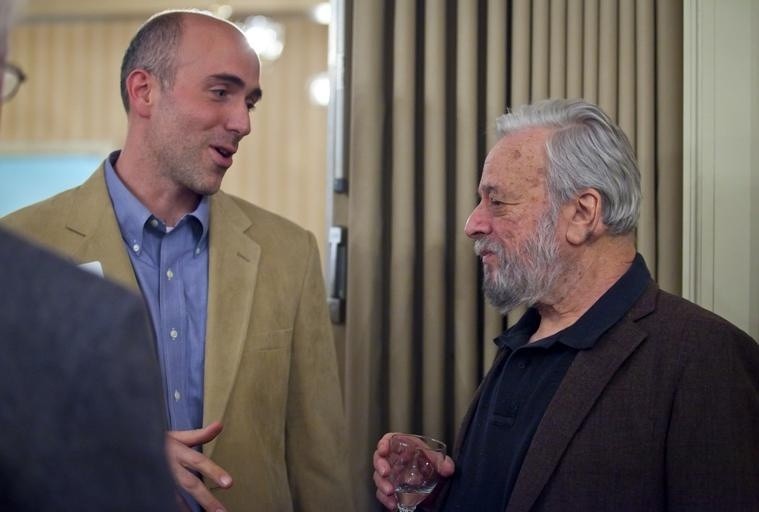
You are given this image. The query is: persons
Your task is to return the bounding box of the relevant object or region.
[0,223,174,510]
[373,95,758,511]
[0,6,359,510]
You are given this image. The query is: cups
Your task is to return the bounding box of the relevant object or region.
[387,433,447,512]
